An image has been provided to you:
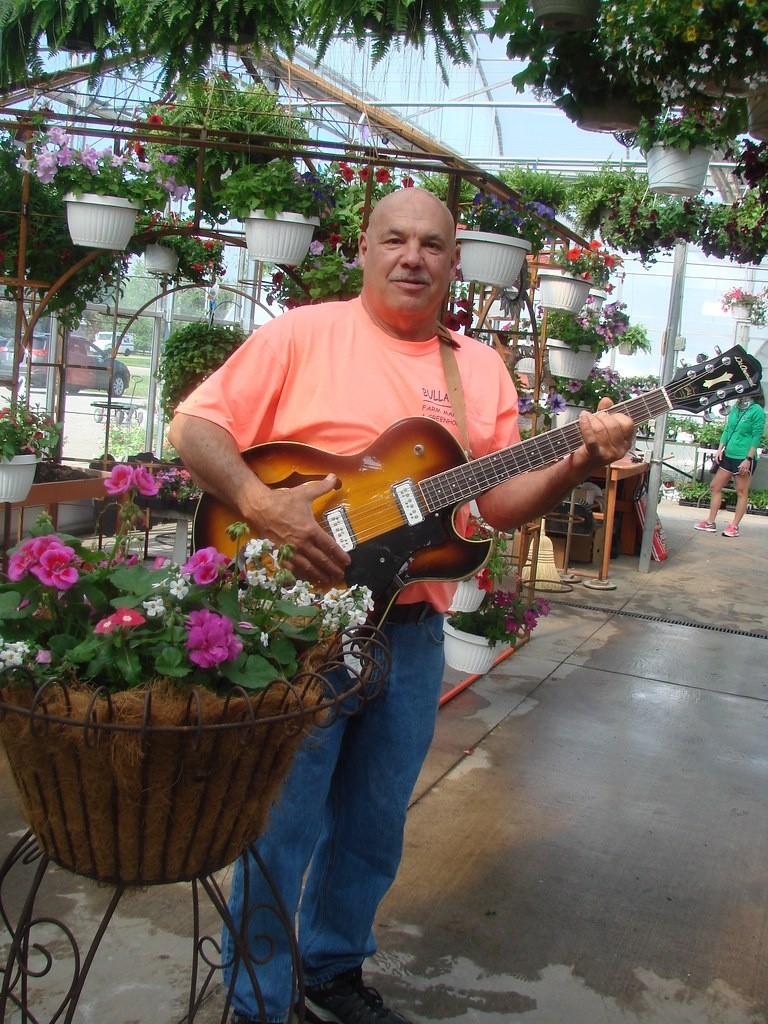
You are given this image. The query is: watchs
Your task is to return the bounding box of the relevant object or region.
[746,457,752,461]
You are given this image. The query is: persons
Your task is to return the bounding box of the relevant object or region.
[167,186,637,1024]
[693,387,766,537]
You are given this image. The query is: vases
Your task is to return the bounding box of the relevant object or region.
[449,577,486,612]
[536,268,594,314]
[583,288,606,312]
[442,615,503,675]
[647,141,713,196]
[746,97,768,141]
[694,74,768,98]
[556,400,591,428]
[245,209,320,265]
[734,305,750,320]
[145,242,178,273]
[0,455,42,503]
[62,192,140,250]
[546,338,598,380]
[456,230,532,288]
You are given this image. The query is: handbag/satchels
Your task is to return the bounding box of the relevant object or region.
[710,452,725,474]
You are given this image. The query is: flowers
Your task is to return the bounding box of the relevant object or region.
[722,287,768,326]
[589,0,768,270]
[0,68,629,694]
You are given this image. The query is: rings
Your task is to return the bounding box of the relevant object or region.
[744,470,747,471]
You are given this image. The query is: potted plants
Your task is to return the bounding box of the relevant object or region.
[619,324,652,355]
[157,321,248,424]
[0,0,487,103]
[489,0,664,242]
[665,416,768,516]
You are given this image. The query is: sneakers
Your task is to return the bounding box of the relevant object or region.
[722,524,739,537]
[693,521,716,533]
[297,967,413,1024]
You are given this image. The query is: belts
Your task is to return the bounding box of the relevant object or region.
[379,603,436,619]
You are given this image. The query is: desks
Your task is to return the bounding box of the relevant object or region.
[589,455,647,580]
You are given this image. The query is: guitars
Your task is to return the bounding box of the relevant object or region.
[190,344,763,674]
